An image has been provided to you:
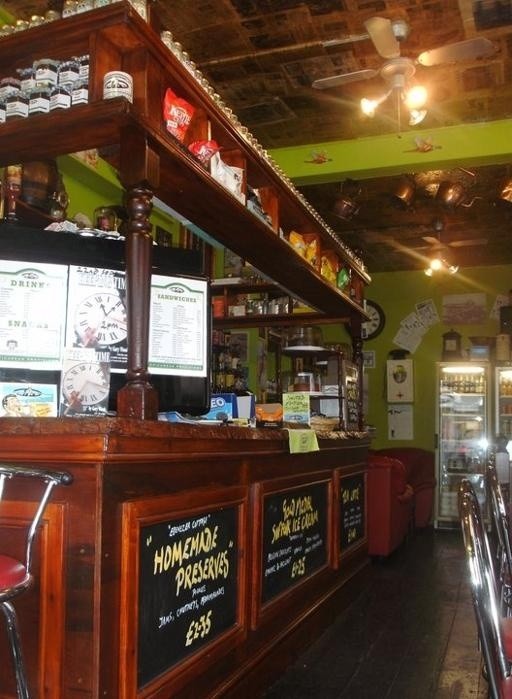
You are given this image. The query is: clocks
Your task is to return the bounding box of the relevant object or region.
[344,300,386,341]
[62,362,111,407]
[73,292,128,347]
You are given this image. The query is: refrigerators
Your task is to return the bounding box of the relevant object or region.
[432,358,511,531]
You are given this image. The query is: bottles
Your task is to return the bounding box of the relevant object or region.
[439,373,486,395]
[499,375,512,439]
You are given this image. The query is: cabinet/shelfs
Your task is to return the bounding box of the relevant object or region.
[275,343,346,433]
[0,2,371,331]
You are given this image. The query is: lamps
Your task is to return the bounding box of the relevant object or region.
[423,251,461,278]
[358,73,428,140]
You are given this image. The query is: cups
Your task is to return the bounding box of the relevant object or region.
[93,205,120,231]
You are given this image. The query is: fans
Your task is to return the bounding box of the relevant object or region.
[393,216,489,254]
[310,14,496,89]
